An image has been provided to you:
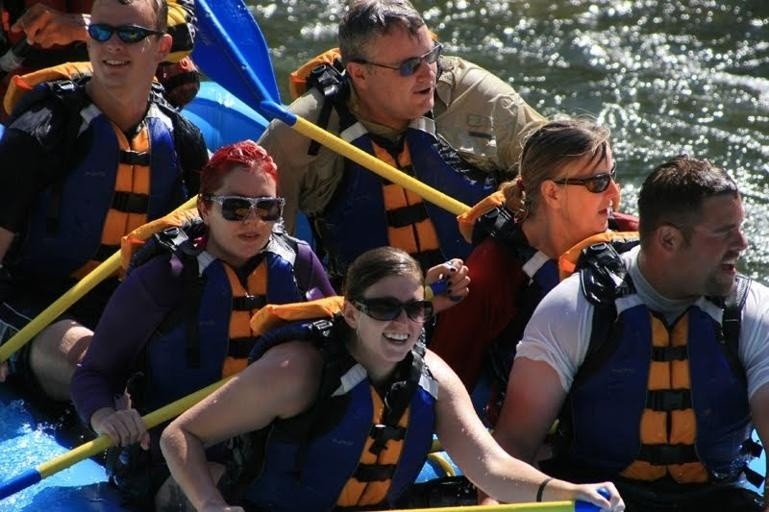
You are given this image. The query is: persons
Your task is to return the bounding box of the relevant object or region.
[67,139,334,511]
[258,0,542,294]
[0,0,199,120]
[427,116,640,430]
[152,248,626,512]
[476,157,769,512]
[0,1,212,402]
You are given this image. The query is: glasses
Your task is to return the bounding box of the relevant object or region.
[353,38,443,76]
[555,160,617,193]
[348,296,434,323]
[203,193,285,223]
[87,22,163,43]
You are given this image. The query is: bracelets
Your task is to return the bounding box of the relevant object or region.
[533,476,557,502]
